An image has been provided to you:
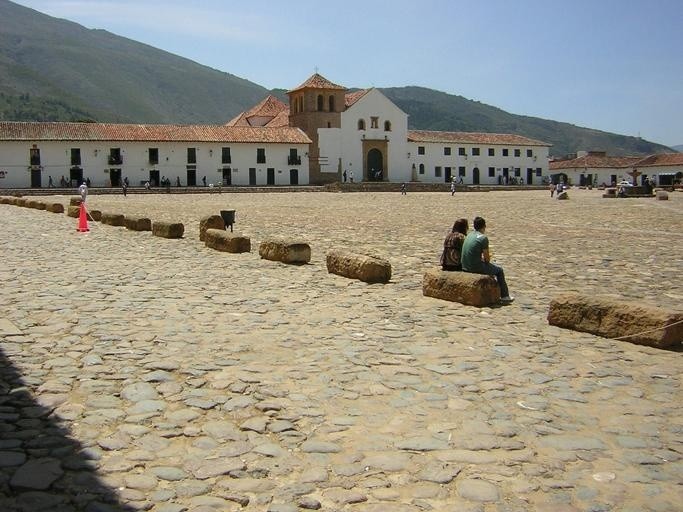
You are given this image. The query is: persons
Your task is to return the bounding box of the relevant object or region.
[460,216,515,303]
[440,218,469,271]
[143,181,151,189]
[350,169,354,184]
[77,176,91,188]
[593,178,631,198]
[343,169,347,183]
[48,175,57,188]
[202,175,206,186]
[543,175,564,198]
[400,183,407,195]
[510,175,524,186]
[118,176,129,196]
[450,174,463,196]
[161,175,181,188]
[643,174,657,194]
[60,175,71,188]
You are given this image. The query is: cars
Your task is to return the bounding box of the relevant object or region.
[617,181,634,187]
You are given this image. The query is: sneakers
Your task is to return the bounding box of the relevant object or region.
[502,295,514,301]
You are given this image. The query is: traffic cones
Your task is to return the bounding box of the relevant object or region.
[77,202,90,231]
